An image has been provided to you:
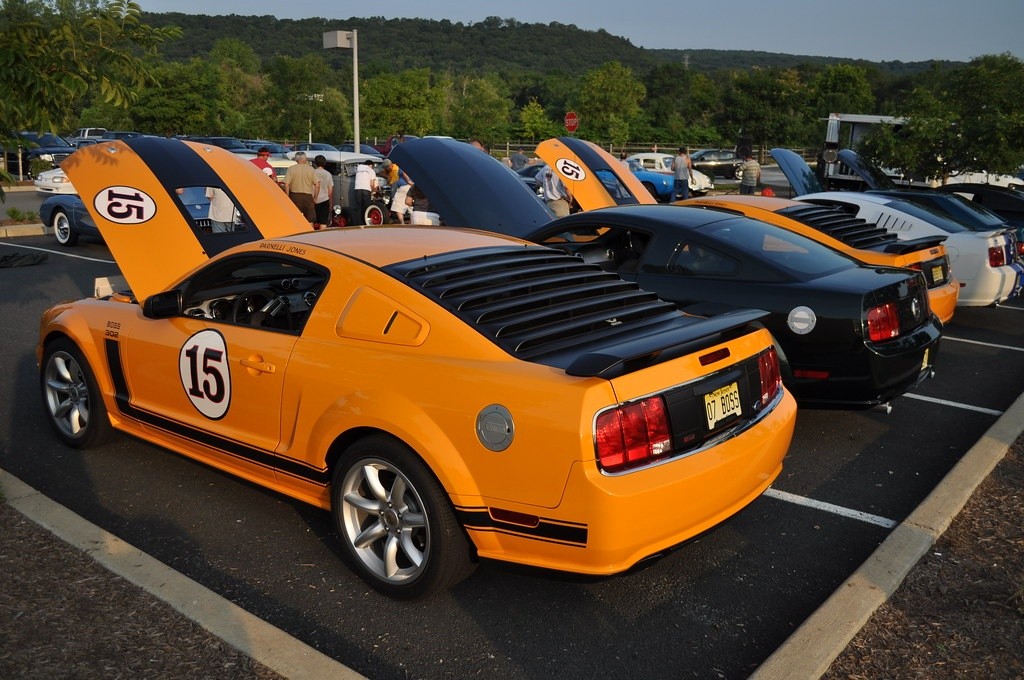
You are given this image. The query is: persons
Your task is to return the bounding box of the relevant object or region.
[739,153,762,195]
[671,147,696,202]
[466,138,573,218]
[175,147,441,233]
[615,152,630,198]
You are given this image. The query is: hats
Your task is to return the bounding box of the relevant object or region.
[382,159,392,166]
[257,151,273,157]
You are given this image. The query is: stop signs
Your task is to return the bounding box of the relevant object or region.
[564,112,578,132]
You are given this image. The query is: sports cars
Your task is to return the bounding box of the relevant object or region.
[836,146,1024,261]
[769,147,1024,307]
[533,136,959,326]
[31,135,799,602]
[385,136,945,411]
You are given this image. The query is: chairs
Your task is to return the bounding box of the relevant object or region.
[677,245,721,272]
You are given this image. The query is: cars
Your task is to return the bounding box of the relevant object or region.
[789,188,1017,307]
[0,117,753,244]
